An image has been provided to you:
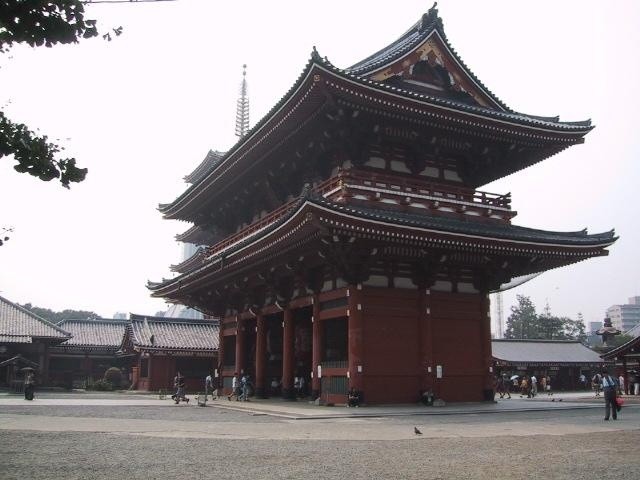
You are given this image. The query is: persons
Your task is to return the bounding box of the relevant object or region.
[494,373,553,399]
[205,372,217,401]
[422,388,434,406]
[24,370,34,400]
[294,374,305,394]
[228,368,254,401]
[172,370,189,404]
[601,368,619,420]
[348,387,359,408]
[579,370,640,397]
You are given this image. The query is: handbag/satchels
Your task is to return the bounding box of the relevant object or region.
[615,396,624,412]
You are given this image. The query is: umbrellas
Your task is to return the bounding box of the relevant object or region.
[19,367,36,371]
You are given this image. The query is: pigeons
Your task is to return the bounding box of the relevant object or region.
[414,426,422,435]
